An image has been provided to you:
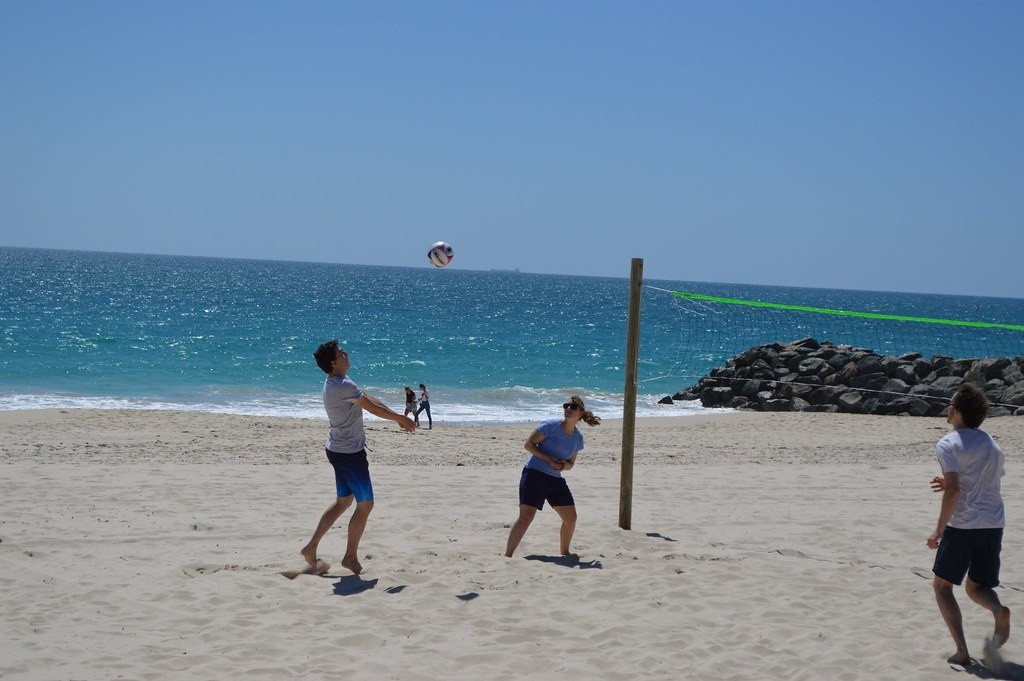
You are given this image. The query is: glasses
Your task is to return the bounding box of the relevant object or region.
[946,400,960,410]
[563,403,579,410]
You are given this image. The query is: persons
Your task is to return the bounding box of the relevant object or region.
[301,340,416,581]
[404,387,420,426]
[927,384,1011,663]
[505,397,601,558]
[414,384,432,429]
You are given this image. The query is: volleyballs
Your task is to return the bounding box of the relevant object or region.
[427,240,454,268]
